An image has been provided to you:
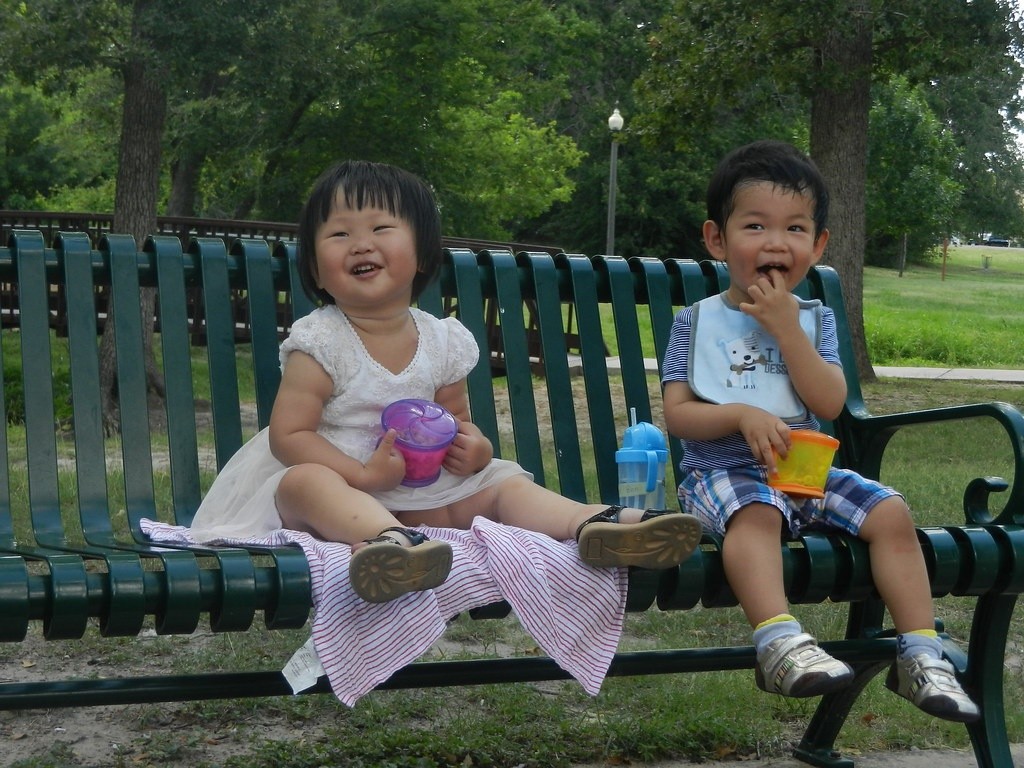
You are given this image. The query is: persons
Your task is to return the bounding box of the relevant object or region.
[662,141,979,722]
[225,161,701,602]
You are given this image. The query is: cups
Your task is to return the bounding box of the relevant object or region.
[375,399,458,489]
[767,429,841,500]
[615,407,670,511]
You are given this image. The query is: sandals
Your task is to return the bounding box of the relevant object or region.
[575,504,702,568]
[349,528,453,605]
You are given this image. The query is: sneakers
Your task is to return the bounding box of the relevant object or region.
[755,632,855,700]
[886,654,981,723]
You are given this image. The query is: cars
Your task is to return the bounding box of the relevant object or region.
[951,230,975,246]
[984,239,1018,248]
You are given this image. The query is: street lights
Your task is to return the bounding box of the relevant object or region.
[605,106,624,256]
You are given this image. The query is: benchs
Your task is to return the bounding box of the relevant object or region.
[0,229,1024,767]
